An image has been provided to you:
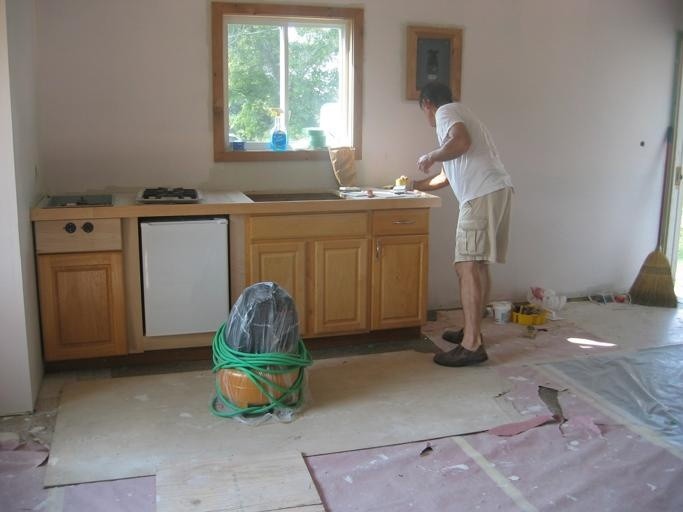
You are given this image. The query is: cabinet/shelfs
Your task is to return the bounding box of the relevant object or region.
[249,212,369,339]
[33,219,129,362]
[372,209,429,328]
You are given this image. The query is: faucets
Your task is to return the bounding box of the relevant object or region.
[494,300,513,324]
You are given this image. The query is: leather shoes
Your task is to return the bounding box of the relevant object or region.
[442,325,482,345]
[433,344,488,367]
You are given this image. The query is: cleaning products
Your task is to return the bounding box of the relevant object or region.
[271,106,287,150]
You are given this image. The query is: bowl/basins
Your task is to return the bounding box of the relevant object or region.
[304,127,326,149]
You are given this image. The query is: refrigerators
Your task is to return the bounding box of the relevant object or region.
[137,218,230,336]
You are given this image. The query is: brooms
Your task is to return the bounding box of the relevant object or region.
[626,126,683,308]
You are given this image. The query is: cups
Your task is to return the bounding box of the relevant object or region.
[231,141,246,151]
[492,304,509,323]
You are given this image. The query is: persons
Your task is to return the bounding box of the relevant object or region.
[407,78,516,369]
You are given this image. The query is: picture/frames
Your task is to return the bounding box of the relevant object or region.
[403,24,463,104]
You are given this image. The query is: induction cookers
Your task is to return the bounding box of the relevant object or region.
[42,194,114,206]
